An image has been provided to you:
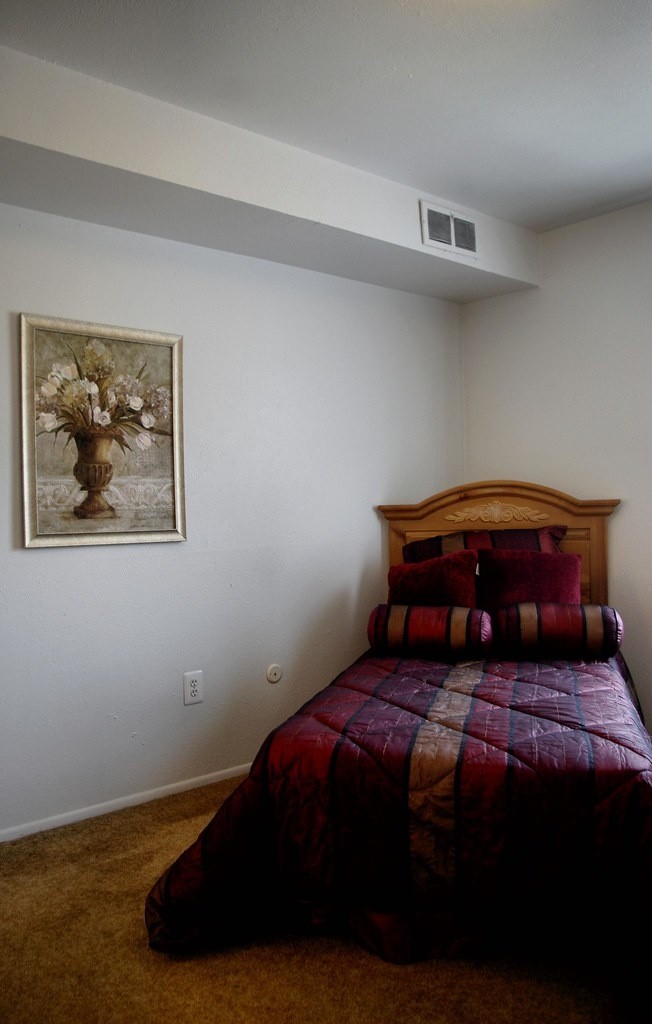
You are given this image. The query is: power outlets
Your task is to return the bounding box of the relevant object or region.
[184,670,205,706]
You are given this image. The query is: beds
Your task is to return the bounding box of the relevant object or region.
[144,480,652,966]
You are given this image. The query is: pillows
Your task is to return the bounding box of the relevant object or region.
[402,524,567,564]
[367,604,493,649]
[388,550,477,608]
[478,546,583,607]
[495,602,622,658]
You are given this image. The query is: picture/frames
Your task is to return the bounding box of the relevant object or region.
[18,312,187,549]
